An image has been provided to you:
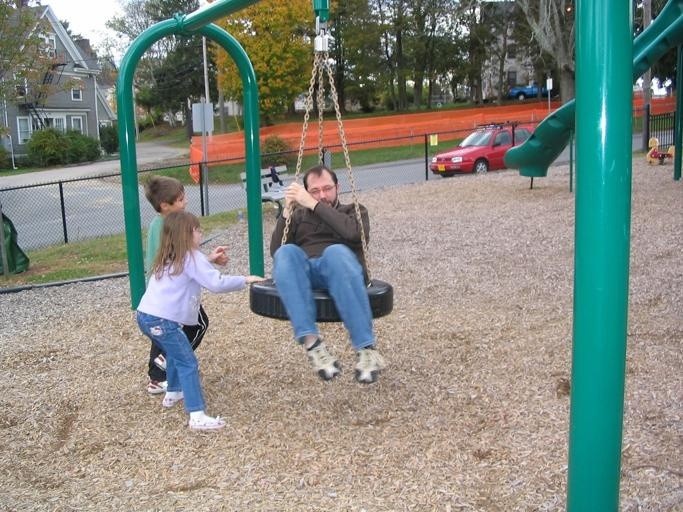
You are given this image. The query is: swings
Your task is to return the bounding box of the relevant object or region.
[251,16,392,320]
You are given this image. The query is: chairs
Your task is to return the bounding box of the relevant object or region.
[238,165,311,220]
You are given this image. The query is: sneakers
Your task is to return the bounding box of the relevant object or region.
[189,416,226,429]
[153,354,166,371]
[354,347,384,384]
[162,392,183,407]
[148,380,168,394]
[304,340,341,381]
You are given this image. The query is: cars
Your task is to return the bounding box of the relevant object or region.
[427,120,535,181]
[509,84,551,102]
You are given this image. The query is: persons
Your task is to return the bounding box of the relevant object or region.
[141,172,230,394]
[136,213,267,429]
[270,166,382,384]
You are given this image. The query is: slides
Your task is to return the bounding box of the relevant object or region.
[503,0,682,176]
